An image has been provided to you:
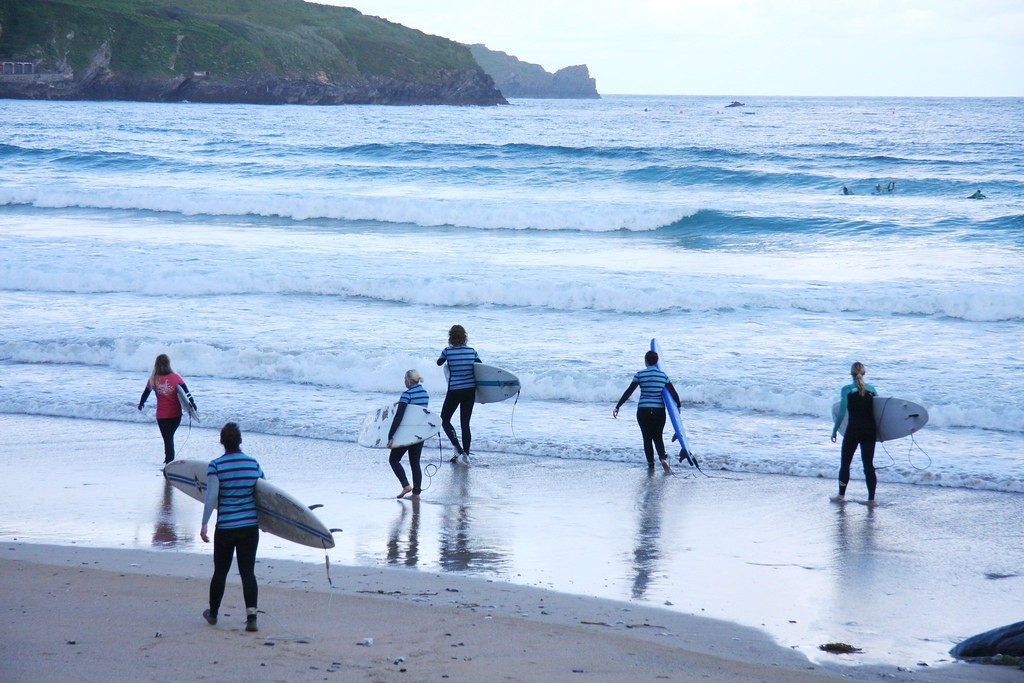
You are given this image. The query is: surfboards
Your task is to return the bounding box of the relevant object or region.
[357,403,445,450]
[830,395,931,443]
[441,362,522,405]
[651,337,700,470]
[174,382,200,423]
[164,459,343,552]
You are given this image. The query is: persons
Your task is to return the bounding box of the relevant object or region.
[613,351,682,470]
[386,370,429,498]
[436,325,482,463]
[969,190,985,199]
[829,362,878,503]
[843,179,896,194]
[200,423,267,631]
[138,354,197,471]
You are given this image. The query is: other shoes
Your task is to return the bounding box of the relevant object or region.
[203,608,218,625]
[245,614,258,631]
